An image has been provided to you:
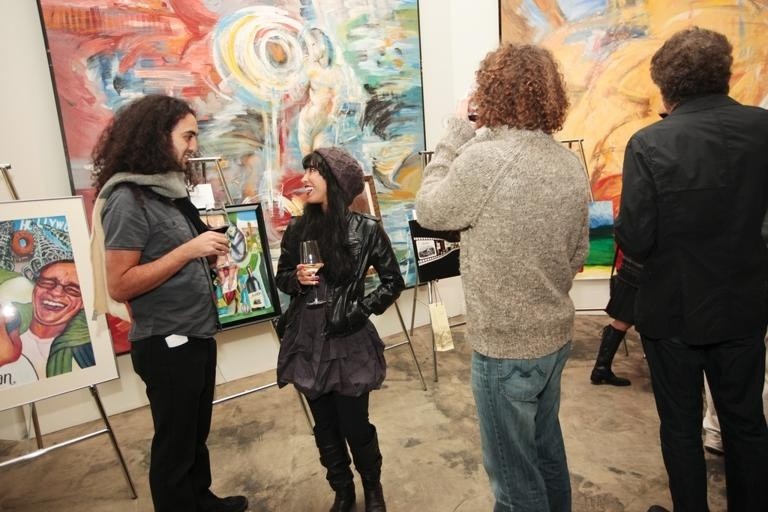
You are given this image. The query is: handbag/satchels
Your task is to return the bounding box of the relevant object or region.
[427,279,455,352]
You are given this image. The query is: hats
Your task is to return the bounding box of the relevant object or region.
[314,148,364,207]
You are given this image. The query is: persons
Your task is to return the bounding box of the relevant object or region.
[613,27,768,512]
[274,147,405,512]
[0,259,84,393]
[91,94,250,512]
[700,373,725,456]
[415,44,591,512]
[590,243,644,387]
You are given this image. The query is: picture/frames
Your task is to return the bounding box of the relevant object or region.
[195,203,283,333]
[37,1,428,358]
[348,176,386,279]
[499,1,768,282]
[0,196,120,412]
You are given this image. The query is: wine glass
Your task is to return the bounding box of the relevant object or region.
[415,238,462,266]
[441,100,480,130]
[205,202,237,270]
[299,239,327,306]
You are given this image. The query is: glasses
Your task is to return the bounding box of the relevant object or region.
[38,278,81,297]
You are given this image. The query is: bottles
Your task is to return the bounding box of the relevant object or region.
[246,266,265,311]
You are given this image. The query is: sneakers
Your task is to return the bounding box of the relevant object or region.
[219,496,248,511]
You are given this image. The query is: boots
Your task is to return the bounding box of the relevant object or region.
[313,427,355,512]
[591,325,631,385]
[346,424,385,512]
